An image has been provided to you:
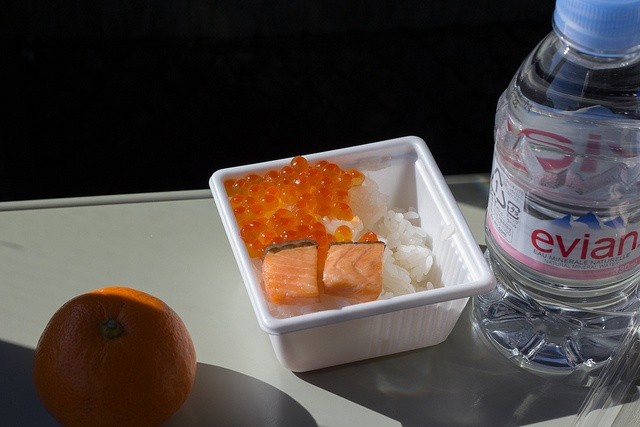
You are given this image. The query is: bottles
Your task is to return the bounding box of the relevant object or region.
[469,0,640,380]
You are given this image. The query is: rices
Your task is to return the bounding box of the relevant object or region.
[324,176,443,311]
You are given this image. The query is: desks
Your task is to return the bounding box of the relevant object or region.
[1,176,632,425]
